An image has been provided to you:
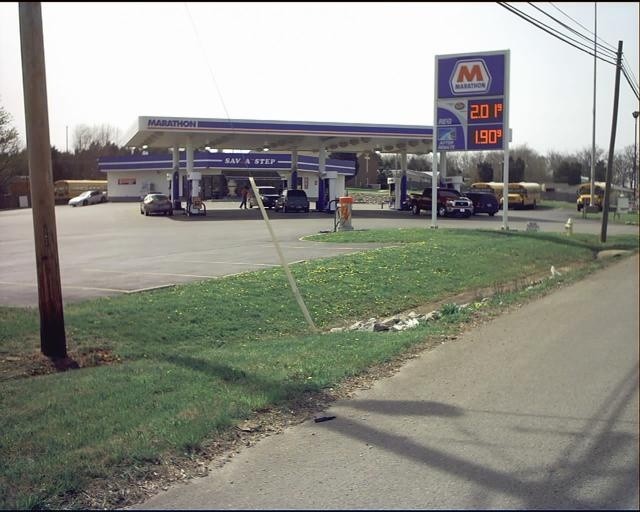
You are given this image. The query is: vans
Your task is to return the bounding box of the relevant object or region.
[463,192,499,216]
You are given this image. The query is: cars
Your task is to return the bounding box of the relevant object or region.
[141,194,173,216]
[406,195,413,211]
[69,191,107,206]
[250,187,309,213]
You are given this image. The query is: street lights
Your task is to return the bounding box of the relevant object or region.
[632,111,640,200]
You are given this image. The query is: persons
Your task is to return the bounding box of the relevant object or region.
[239,185,251,209]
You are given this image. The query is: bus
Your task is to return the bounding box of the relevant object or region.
[472,182,541,209]
[577,182,615,212]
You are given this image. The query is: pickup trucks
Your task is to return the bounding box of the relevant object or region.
[413,189,473,217]
